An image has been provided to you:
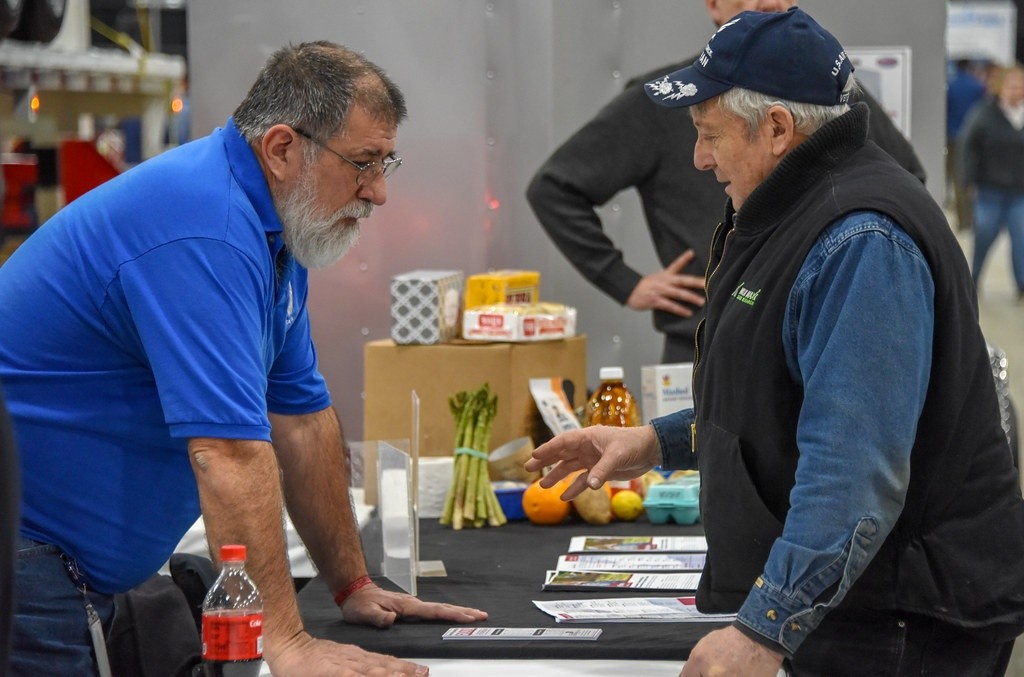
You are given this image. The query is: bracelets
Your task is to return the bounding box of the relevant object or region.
[334,575,372,606]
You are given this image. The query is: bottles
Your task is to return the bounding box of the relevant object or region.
[201,544,265,676]
[584,367,648,522]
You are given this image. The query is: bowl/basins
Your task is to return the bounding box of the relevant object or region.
[486,481,530,520]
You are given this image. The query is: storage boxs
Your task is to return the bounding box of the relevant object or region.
[362,332,589,504]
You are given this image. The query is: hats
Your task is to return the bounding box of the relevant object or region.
[644,6,856,108]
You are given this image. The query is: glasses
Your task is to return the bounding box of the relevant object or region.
[290,126,402,187]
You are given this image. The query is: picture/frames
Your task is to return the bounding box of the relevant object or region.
[841,45,912,142]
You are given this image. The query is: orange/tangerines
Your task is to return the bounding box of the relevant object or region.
[523,467,644,524]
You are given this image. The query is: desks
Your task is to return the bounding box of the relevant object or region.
[294,512,787,677]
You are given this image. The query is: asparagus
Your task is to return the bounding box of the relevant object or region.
[440,382,508,527]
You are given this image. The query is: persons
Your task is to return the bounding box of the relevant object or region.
[0,39,487,676]
[525,0,927,363]
[963,64,1024,309]
[526,6,1024,674]
[945,57,986,230]
[959,63,1006,207]
